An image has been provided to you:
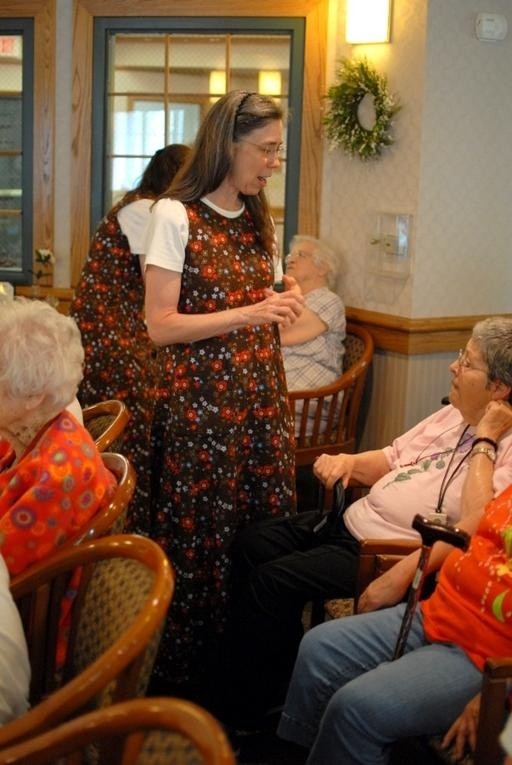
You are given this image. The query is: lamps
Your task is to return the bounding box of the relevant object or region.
[345,0,390,44]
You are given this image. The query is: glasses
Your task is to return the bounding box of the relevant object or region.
[283,250,307,264]
[237,137,285,160]
[457,348,499,380]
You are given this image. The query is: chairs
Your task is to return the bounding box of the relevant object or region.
[377,553,512,765]
[316,456,421,619]
[80,399,130,451]
[0,532,176,744]
[284,321,372,514]
[0,697,235,765]
[59,448,136,550]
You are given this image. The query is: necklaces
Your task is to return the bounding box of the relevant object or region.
[398,418,475,467]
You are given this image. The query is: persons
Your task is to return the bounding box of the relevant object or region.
[1,295,119,674]
[274,484,510,764]
[67,143,195,538]
[139,90,304,714]
[205,316,510,764]
[1,558,36,737]
[266,232,351,436]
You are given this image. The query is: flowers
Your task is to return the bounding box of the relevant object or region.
[319,53,400,164]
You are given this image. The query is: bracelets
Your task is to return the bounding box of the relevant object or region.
[471,437,497,452]
[469,448,497,465]
